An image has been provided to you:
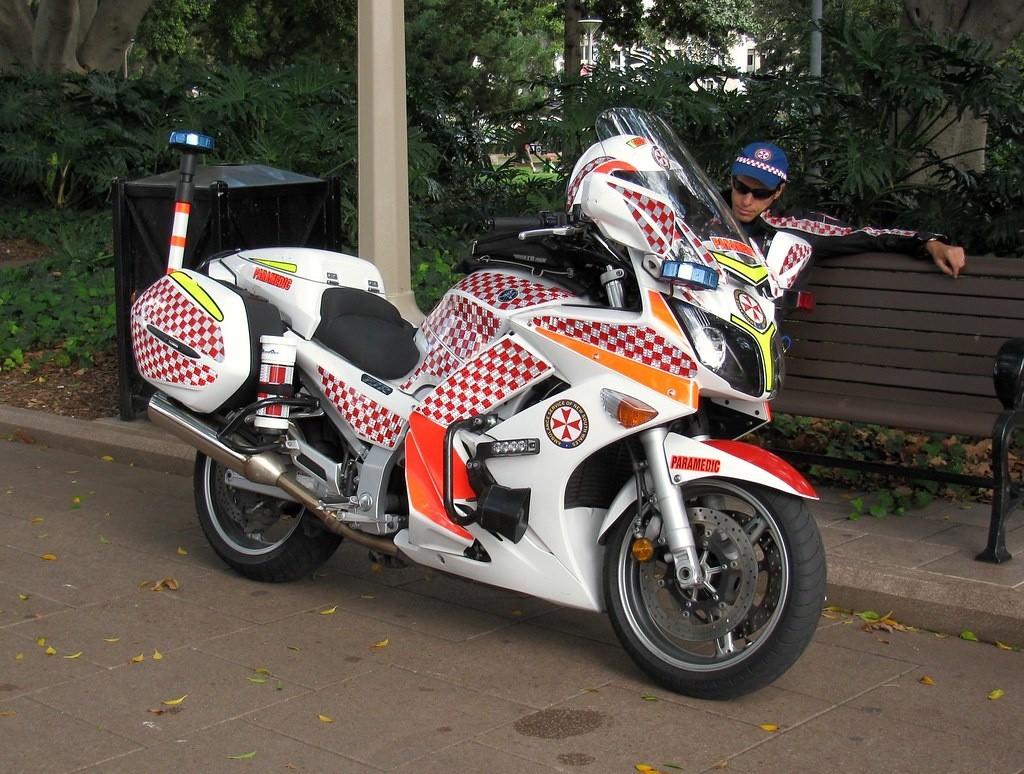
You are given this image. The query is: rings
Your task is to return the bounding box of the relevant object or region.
[960,258,964,261]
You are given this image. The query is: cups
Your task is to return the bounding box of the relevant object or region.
[254,336,298,429]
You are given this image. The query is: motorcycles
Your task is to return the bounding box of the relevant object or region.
[130,106,830,702]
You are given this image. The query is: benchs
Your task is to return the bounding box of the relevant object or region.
[700,251,1024,564]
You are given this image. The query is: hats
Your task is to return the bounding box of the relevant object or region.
[732,143,788,188]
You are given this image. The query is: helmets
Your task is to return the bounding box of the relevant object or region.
[565,134,684,258]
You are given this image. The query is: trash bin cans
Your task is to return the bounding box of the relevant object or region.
[110,162,344,425]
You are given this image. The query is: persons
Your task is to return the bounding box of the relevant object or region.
[704,143,965,321]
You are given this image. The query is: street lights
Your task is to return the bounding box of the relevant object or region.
[578,13,605,85]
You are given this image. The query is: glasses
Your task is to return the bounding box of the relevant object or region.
[733,175,780,199]
